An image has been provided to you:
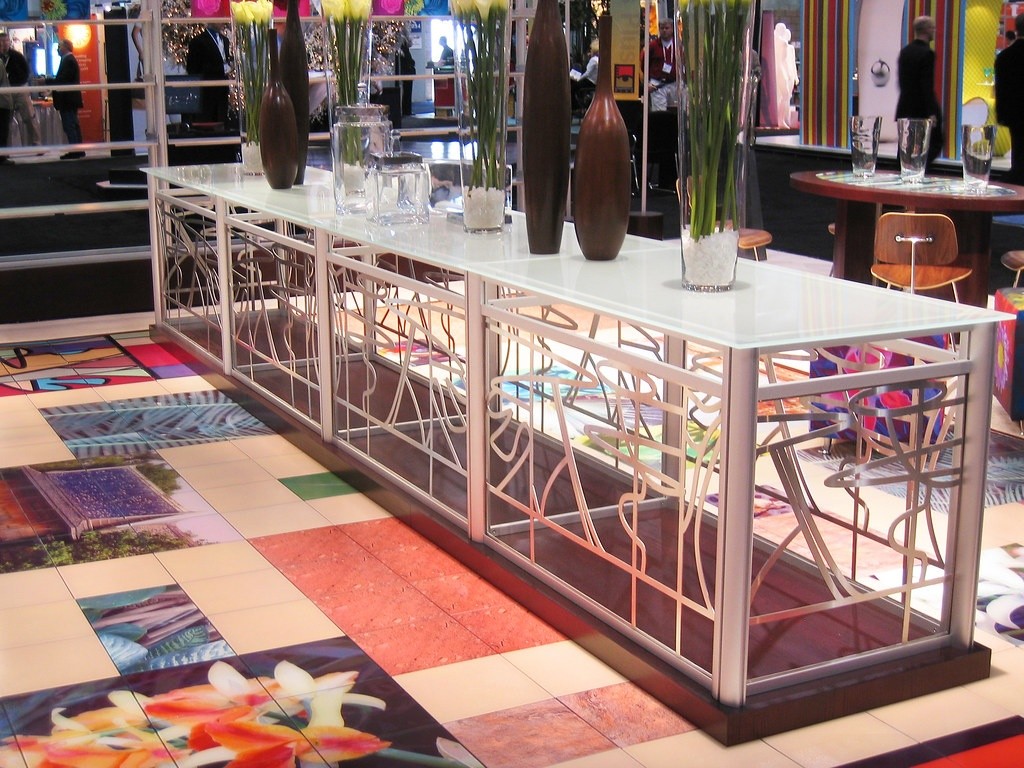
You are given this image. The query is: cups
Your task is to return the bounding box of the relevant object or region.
[848,116,882,177]
[897,118,932,184]
[961,124,996,189]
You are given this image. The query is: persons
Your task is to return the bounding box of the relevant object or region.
[0,32,46,164]
[509,20,529,86]
[95,0,141,10]
[400,37,416,116]
[752,49,760,69]
[640,17,676,91]
[570,38,599,110]
[36,39,86,159]
[131,0,162,136]
[774,23,799,129]
[186,23,235,129]
[894,15,944,173]
[426,36,453,66]
[994,13,1024,185]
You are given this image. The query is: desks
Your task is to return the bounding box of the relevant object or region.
[139,161,1018,748]
[788,169,1024,346]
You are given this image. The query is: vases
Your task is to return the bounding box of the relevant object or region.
[259,29,299,190]
[672,0,756,293]
[450,0,513,235]
[572,16,632,261]
[278,0,309,186]
[522,0,571,254]
[229,0,275,176]
[319,0,374,204]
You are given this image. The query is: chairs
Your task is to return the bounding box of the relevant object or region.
[869,211,974,352]
[674,175,773,262]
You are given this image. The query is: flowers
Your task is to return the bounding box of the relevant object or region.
[321,0,372,166]
[452,0,514,200]
[230,0,273,147]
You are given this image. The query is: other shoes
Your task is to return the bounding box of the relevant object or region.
[0,158,16,166]
[60,149,87,159]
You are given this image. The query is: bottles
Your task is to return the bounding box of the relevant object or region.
[333,82,429,227]
